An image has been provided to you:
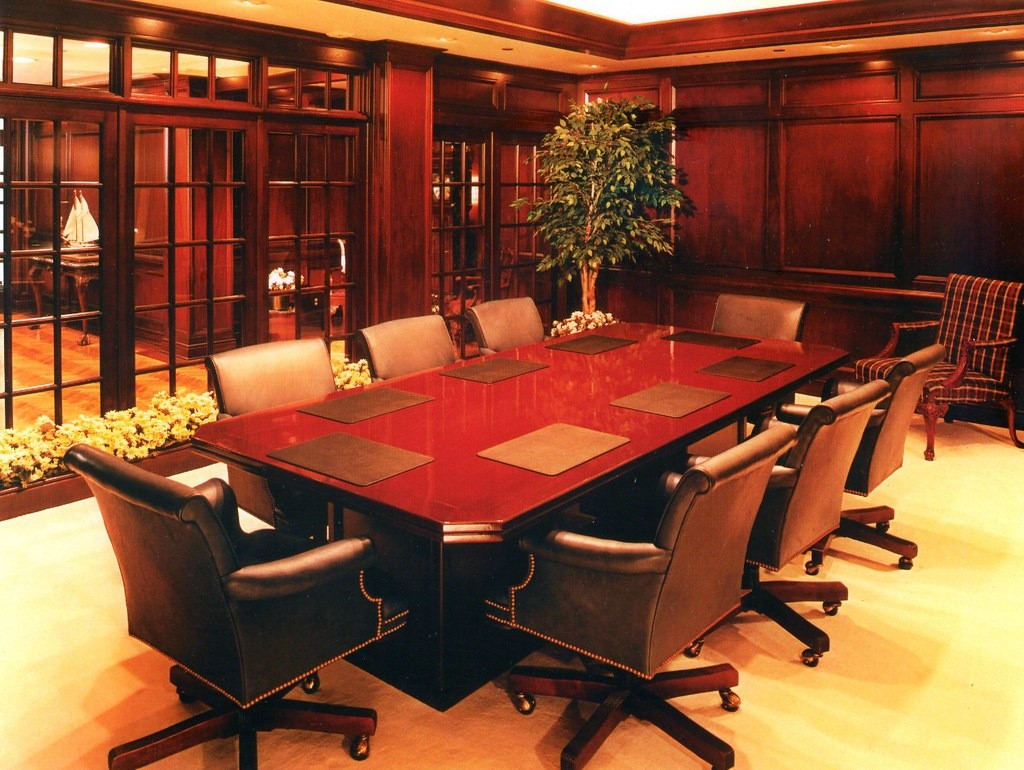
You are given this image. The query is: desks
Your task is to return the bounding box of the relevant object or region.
[28,252,100,345]
[189,320,851,712]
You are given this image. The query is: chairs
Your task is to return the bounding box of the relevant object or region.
[62,444,410,770]
[465,298,551,355]
[482,424,801,770]
[711,295,811,440]
[358,316,462,381]
[754,342,948,575]
[205,338,338,535]
[602,379,890,668]
[854,274,1024,461]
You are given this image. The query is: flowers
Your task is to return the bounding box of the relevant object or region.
[551,310,619,336]
[331,352,374,391]
[269,268,304,290]
[0,387,219,490]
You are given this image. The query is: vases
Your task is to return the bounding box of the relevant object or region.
[0,444,220,522]
[272,287,289,311]
